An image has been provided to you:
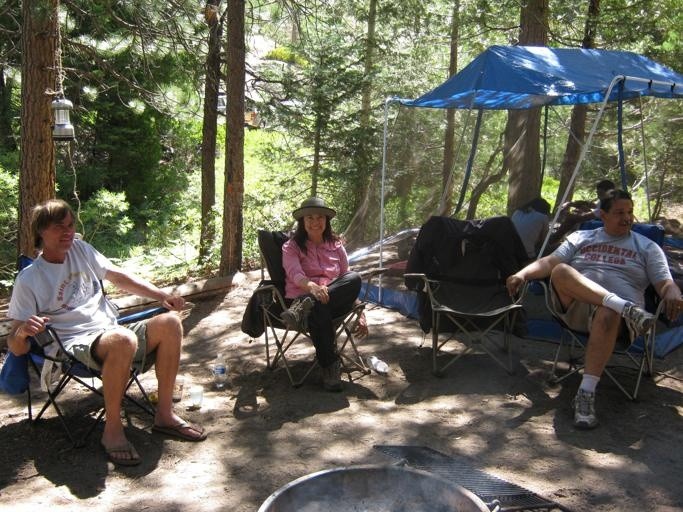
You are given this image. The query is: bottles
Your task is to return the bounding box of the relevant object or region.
[143,391,158,403]
[366,354,390,375]
[212,353,226,388]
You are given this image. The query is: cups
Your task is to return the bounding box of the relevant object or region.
[189,384,204,408]
[172,378,184,400]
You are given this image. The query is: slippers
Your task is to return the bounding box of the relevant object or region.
[151,416,207,441]
[95,436,141,465]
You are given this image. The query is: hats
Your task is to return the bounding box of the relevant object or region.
[293,196,337,221]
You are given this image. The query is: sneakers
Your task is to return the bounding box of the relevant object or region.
[571,389,600,429]
[280,297,316,331]
[321,358,344,392]
[620,301,657,334]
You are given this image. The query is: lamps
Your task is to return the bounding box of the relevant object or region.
[44,0,85,241]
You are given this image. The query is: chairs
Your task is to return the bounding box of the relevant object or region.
[538,220,667,404]
[257,230,371,389]
[403,215,535,377]
[17,254,170,449]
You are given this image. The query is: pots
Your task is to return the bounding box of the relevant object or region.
[256,459,505,511]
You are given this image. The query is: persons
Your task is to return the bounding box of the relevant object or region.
[503,187,682,431]
[7,198,209,465]
[567,180,614,220]
[508,196,552,263]
[279,195,363,394]
